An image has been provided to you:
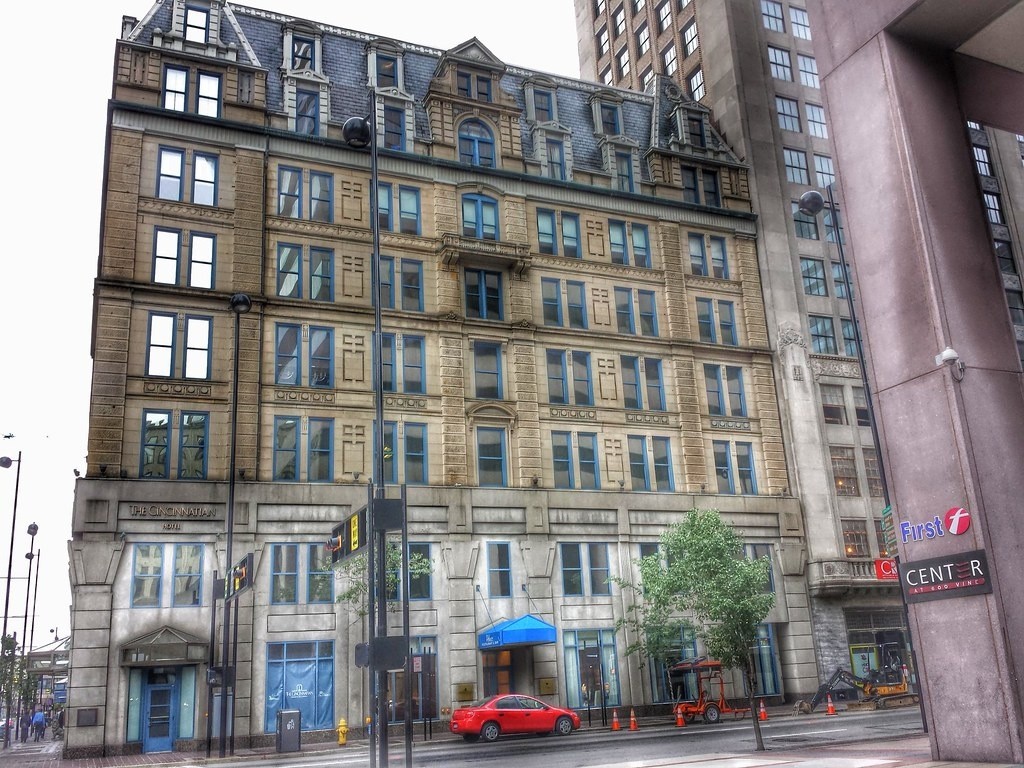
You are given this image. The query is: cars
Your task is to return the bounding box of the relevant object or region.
[450,691,582,741]
[3,718,15,728]
[0,721,6,738]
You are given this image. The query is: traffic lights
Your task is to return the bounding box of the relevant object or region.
[325,535,342,552]
[232,567,246,580]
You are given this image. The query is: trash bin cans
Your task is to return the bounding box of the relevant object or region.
[276,708,301,753]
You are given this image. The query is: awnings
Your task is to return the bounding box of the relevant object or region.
[478,614,557,651]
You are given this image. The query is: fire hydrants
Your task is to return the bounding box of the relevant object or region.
[336,717,350,746]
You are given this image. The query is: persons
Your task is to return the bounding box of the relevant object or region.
[32,707,46,742]
[57,707,63,727]
[19,711,32,743]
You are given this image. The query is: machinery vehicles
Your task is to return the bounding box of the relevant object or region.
[792,642,922,716]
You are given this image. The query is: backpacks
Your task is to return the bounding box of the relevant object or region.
[21,716,30,728]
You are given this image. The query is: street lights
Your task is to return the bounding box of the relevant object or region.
[0,452,21,659]
[219,291,255,755]
[25,549,40,711]
[799,186,929,737]
[344,110,389,768]
[13,522,39,738]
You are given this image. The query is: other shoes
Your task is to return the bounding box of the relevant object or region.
[37,740,38,742]
[21,741,23,742]
[24,739,26,742]
[34,739,36,741]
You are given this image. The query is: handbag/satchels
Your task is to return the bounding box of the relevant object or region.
[34,721,43,730]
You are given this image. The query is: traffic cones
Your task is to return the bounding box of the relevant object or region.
[675,704,688,728]
[627,707,640,731]
[824,694,837,715]
[757,699,770,721]
[609,708,623,731]
